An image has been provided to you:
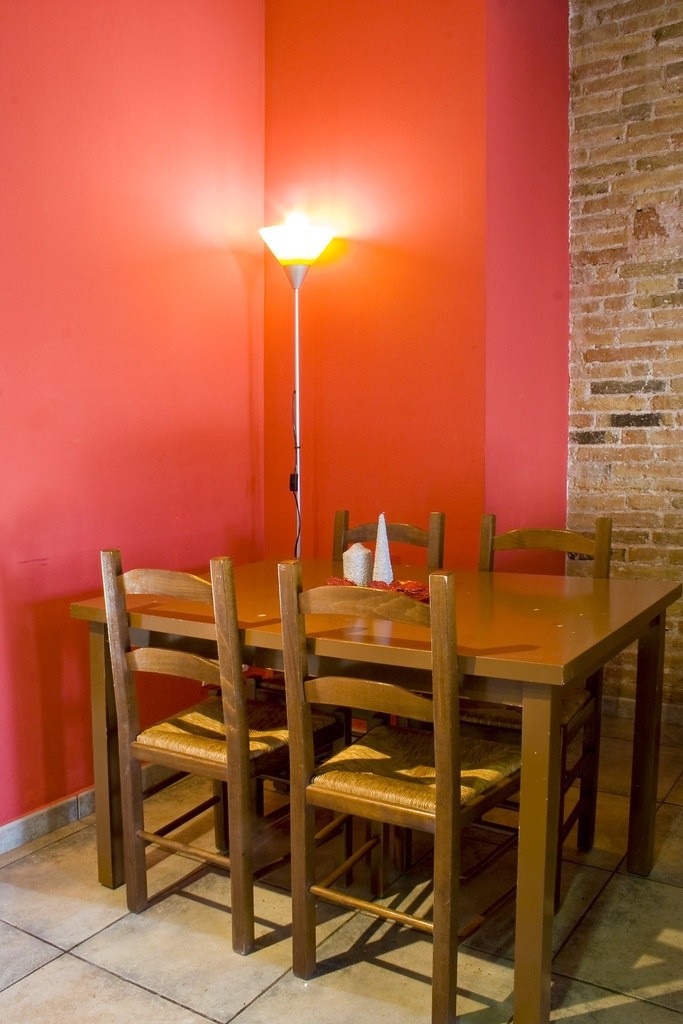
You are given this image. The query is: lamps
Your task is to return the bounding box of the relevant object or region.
[258,225,339,559]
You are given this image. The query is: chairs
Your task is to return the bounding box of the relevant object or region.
[100,547,353,956]
[244,508,446,870]
[396,514,612,917]
[277,558,521,1024]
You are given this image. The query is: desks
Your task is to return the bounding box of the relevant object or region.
[69,558,683,1024]
